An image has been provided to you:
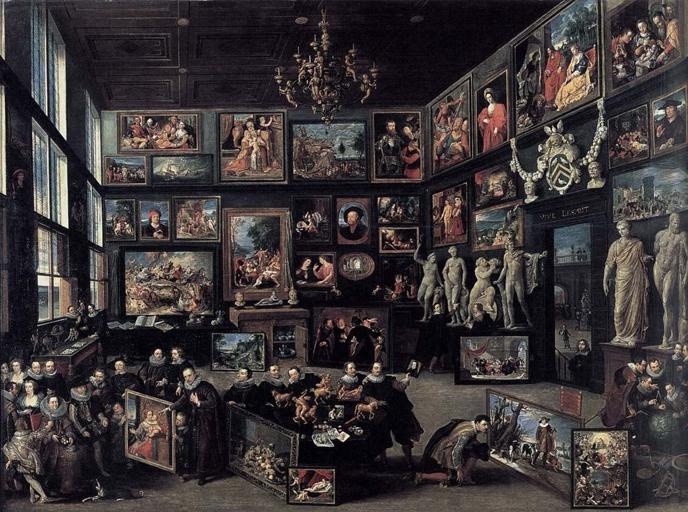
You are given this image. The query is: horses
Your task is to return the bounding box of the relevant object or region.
[355,401,378,420]
[293,403,318,424]
[272,390,293,407]
[314,378,331,405]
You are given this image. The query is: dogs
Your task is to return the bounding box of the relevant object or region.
[546,453,562,472]
[520,442,537,465]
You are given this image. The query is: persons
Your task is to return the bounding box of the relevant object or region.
[0,1,688,505]
